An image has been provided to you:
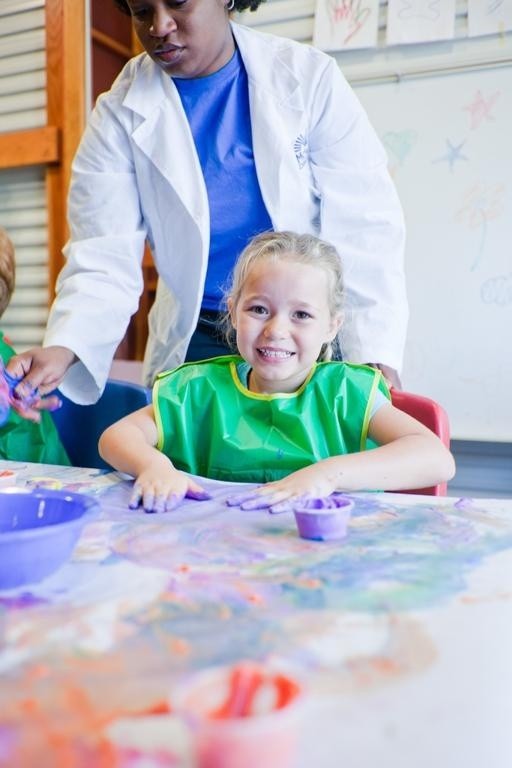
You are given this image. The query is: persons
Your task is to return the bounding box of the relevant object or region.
[4,0,407,422]
[98,231,456,513]
[0,224,73,466]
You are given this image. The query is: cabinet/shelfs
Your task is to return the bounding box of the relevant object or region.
[1,0,163,376]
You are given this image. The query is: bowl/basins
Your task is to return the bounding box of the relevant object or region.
[292,497,354,542]
[0,487,103,598]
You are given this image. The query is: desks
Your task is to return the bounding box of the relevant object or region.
[0,454,512,767]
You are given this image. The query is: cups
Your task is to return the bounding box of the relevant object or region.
[170,661,305,768]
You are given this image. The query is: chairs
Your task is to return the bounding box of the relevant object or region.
[380,390,451,495]
[39,380,155,475]
